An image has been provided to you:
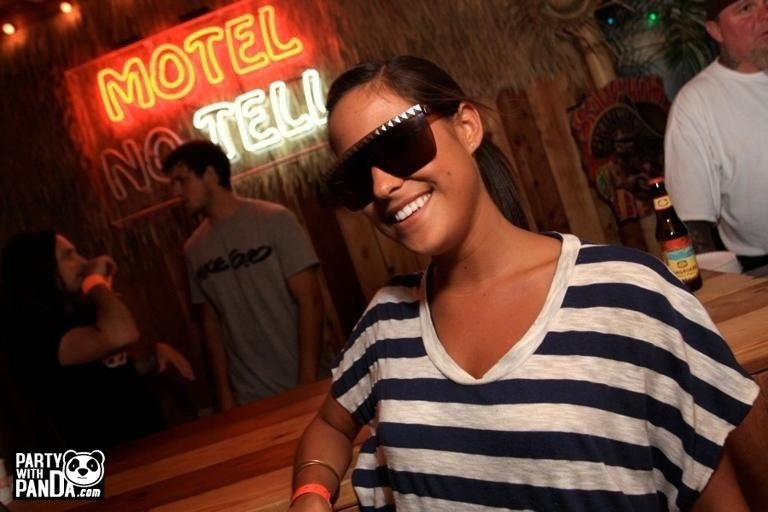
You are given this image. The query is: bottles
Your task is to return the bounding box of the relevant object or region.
[648,178,702,292]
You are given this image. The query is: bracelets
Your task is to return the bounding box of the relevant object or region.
[289,483,336,511]
[294,459,341,500]
[80,273,113,296]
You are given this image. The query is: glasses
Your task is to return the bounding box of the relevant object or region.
[320,104,438,212]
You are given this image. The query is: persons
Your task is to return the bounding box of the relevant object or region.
[2,224,198,460]
[665,0,768,268]
[163,138,333,411]
[288,54,764,511]
